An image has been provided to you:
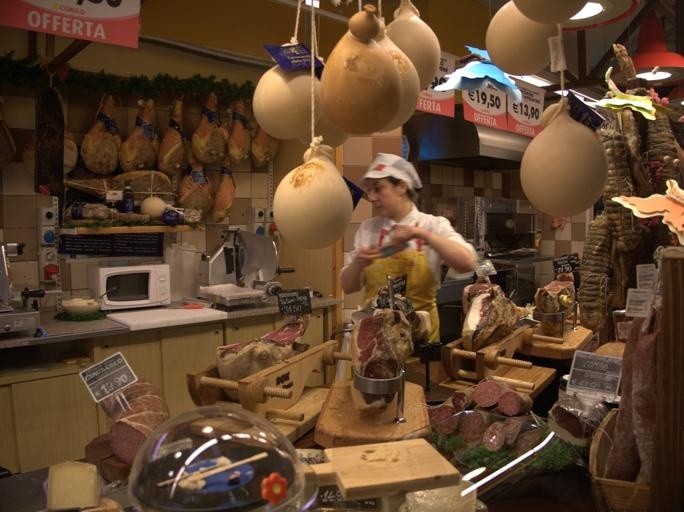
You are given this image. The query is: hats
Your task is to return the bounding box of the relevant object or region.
[361,152,424,193]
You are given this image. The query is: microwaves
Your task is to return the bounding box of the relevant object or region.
[87,263,171,310]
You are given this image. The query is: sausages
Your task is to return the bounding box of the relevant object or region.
[430,379,544,453]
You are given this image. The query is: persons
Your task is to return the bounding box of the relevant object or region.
[336,153,478,377]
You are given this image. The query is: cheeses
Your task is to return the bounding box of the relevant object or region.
[45,462,100,511]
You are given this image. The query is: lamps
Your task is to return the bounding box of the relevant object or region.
[562,0,684,88]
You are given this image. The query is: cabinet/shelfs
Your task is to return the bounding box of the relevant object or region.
[277,306,325,388]
[100,330,163,432]
[162,312,276,421]
[0,374,98,474]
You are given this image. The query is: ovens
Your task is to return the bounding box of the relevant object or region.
[484,212,535,256]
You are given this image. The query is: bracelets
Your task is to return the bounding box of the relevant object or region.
[424,231,435,244]
